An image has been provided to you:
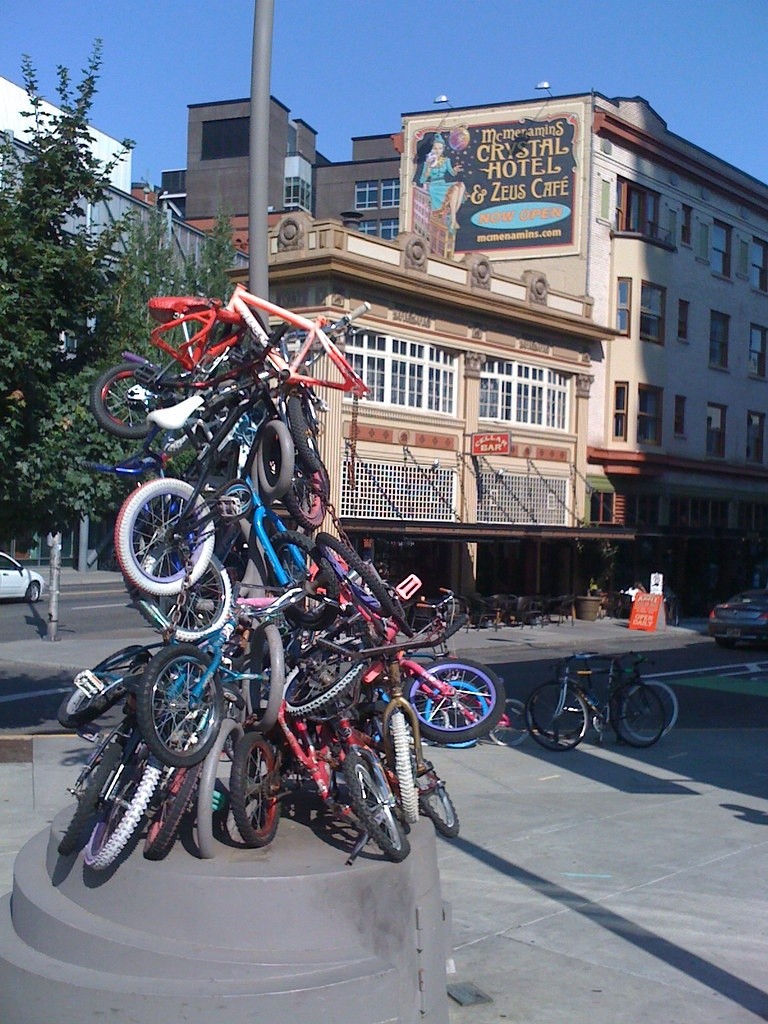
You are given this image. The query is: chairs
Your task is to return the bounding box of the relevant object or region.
[410,593,633,633]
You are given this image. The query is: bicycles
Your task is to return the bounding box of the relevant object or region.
[39,284,531,876]
[532,653,679,742]
[527,649,667,754]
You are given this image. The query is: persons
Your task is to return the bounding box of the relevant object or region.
[629,581,647,602]
[662,577,680,626]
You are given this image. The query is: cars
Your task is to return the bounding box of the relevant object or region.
[0,552,47,602]
[709,589,767,647]
